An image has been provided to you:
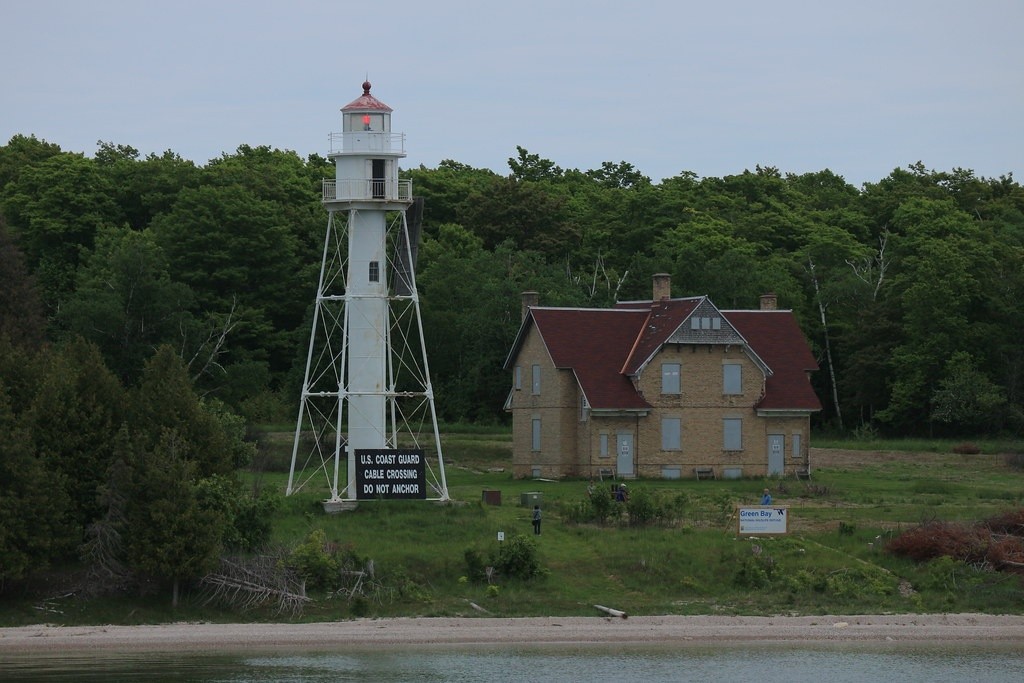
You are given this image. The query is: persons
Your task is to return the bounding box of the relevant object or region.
[759,489,772,505]
[587,480,597,503]
[616,484,631,503]
[532,506,542,535]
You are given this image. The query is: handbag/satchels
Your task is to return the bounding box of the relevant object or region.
[532,520,536,525]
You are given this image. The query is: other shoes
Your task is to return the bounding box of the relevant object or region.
[538,534,541,536]
